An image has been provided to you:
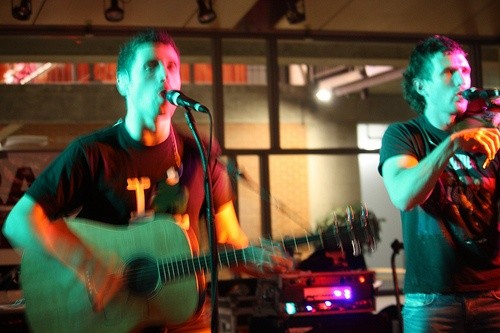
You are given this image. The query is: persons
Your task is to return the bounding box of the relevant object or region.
[2,30,294,333]
[376,34,500,333]
[2,164,37,253]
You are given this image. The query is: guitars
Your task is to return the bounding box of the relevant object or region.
[20,206,382,333]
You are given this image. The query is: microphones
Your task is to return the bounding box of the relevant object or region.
[462,89,500,101]
[166,90,210,113]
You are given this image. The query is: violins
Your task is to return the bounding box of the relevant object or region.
[453,86,500,153]
